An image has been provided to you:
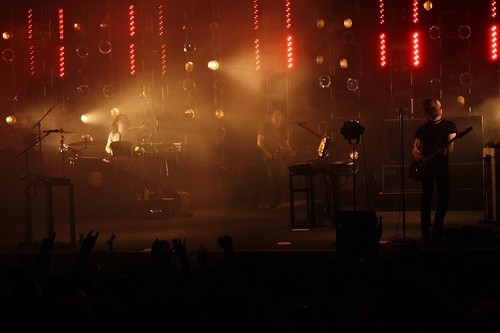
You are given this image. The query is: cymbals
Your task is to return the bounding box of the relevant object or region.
[127,124,155,129]
[68,141,105,148]
[43,129,74,134]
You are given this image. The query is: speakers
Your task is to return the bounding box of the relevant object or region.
[380,114,486,192]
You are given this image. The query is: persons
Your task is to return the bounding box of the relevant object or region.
[412,96,457,246]
[318,128,341,212]
[106,113,133,157]
[257,109,291,208]
[0,221,500,333]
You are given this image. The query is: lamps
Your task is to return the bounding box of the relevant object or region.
[206,49,225,73]
[184,60,197,74]
[71,46,89,58]
[180,20,195,35]
[308,14,361,93]
[422,0,477,98]
[176,40,197,55]
[1,113,17,126]
[0,45,18,64]
[77,111,90,126]
[105,106,121,118]
[97,39,113,55]
[183,78,196,92]
[76,82,90,98]
[97,18,110,32]
[71,16,85,34]
[102,81,116,98]
[0,30,13,41]
[5,88,20,103]
[209,19,222,33]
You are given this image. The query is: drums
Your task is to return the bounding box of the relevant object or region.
[110,140,132,157]
[75,157,119,201]
[58,148,82,173]
[134,144,154,152]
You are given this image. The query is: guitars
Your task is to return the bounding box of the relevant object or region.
[406,126,474,186]
[256,146,306,167]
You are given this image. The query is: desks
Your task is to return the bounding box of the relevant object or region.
[24,182,76,244]
[288,173,341,231]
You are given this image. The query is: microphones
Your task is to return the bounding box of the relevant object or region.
[400,107,407,113]
[139,84,143,99]
[292,120,306,124]
[42,129,58,133]
[67,98,72,104]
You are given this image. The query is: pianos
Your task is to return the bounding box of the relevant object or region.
[288,158,349,229]
